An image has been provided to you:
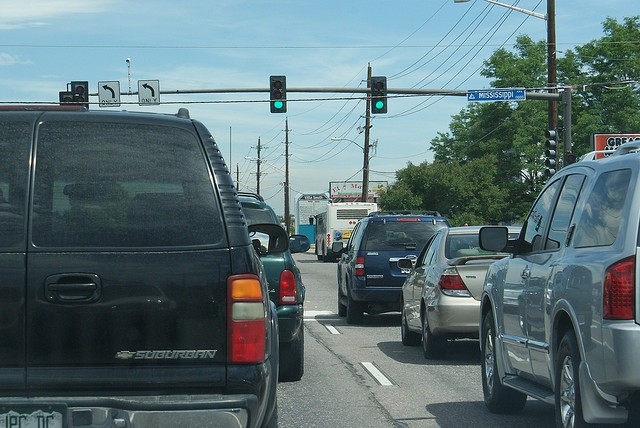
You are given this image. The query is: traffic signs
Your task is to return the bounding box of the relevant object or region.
[99,82,118,109]
[139,80,162,104]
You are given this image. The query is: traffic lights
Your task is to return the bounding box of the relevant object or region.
[540,131,555,179]
[460,0,558,172]
[71,81,87,107]
[242,151,291,225]
[60,90,71,104]
[268,75,287,113]
[330,135,371,201]
[369,76,388,114]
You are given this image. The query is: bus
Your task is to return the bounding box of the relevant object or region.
[309,202,377,264]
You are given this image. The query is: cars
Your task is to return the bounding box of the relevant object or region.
[401,225,524,362]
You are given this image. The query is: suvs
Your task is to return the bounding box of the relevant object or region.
[337,210,453,324]
[479,142,637,428]
[0,104,280,428]
